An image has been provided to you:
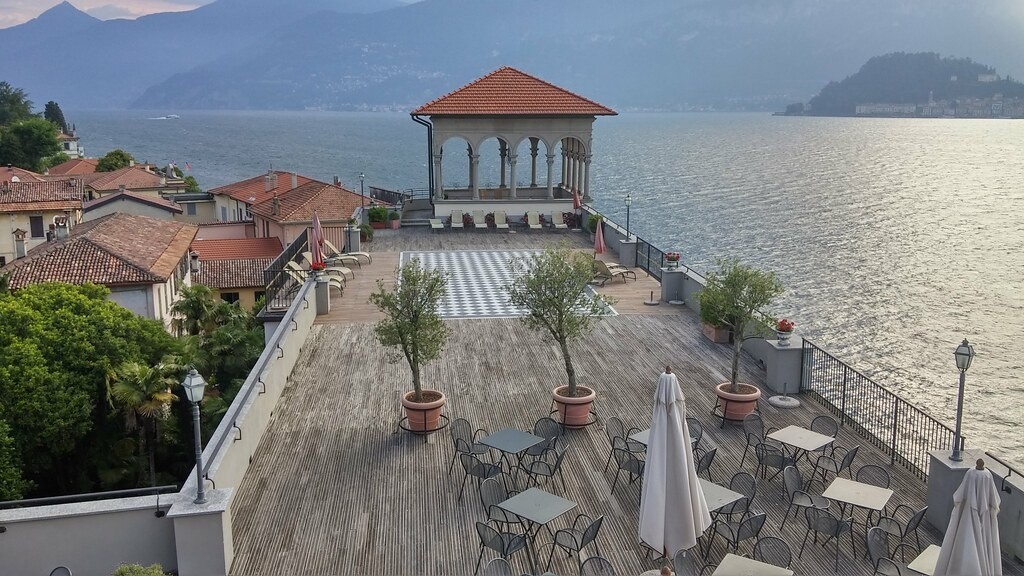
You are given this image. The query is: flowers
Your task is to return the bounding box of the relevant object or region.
[310,262,326,272]
[665,252,680,261]
[777,319,794,332]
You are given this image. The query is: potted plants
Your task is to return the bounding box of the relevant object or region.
[389,212,400,229]
[498,237,621,428]
[363,257,455,436]
[587,214,604,243]
[357,224,373,242]
[366,206,388,229]
[690,256,788,426]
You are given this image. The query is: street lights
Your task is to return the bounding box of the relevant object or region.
[180,365,209,505]
[358,171,365,208]
[947,336,976,462]
[623,193,632,243]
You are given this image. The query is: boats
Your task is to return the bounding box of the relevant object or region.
[166,113,180,119]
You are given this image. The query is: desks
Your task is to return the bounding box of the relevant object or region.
[698,478,745,558]
[907,544,941,576]
[822,477,894,560]
[477,427,545,489]
[498,487,573,566]
[711,553,794,576]
[628,428,698,482]
[768,425,835,483]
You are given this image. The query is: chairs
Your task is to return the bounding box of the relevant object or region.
[472,210,489,233]
[428,219,444,233]
[524,211,544,234]
[447,417,929,576]
[492,211,509,233]
[284,240,372,297]
[548,210,569,234]
[580,250,636,287]
[450,210,464,233]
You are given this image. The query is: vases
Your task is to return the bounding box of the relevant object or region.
[776,330,793,346]
[315,269,325,281]
[666,260,677,270]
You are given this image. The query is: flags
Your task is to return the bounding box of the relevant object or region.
[186,162,190,169]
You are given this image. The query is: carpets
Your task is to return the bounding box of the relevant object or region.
[397,251,619,321]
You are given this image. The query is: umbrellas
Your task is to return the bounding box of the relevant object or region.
[572,188,582,232]
[639,366,712,576]
[314,210,325,246]
[934,459,1002,576]
[593,218,606,270]
[312,229,323,288]
